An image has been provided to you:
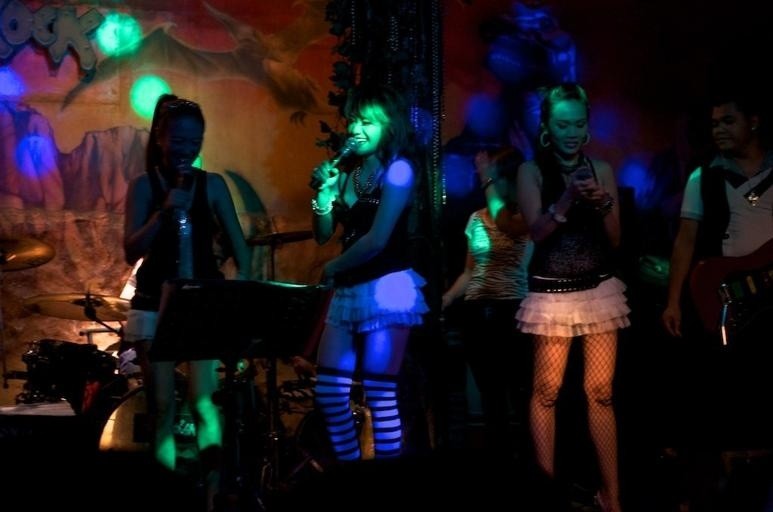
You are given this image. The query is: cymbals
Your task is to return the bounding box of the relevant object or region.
[22,290,132,322]
[252,229,313,247]
[1,231,53,272]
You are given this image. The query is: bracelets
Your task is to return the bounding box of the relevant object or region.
[311,194,334,216]
[594,192,615,215]
[548,205,568,223]
[479,177,495,192]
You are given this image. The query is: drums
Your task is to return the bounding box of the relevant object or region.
[96,379,162,488]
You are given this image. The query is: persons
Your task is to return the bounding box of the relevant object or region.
[441,141,537,462]
[515,82,633,511]
[662,90,772,339]
[309,85,432,463]
[124,91,253,511]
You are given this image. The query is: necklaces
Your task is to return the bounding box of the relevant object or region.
[737,159,764,206]
[553,153,578,168]
[352,164,381,197]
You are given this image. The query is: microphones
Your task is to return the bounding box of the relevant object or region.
[171,174,193,219]
[309,136,357,191]
[575,166,592,181]
